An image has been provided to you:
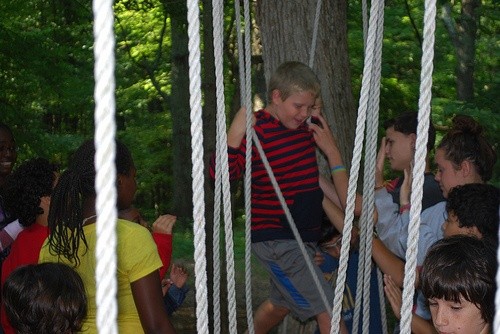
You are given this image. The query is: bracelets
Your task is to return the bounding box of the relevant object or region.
[374,185,387,191]
[331,166,348,175]
[399,204,411,214]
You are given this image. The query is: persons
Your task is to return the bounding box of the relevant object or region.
[0,124,189,334]
[209,61,500,334]
